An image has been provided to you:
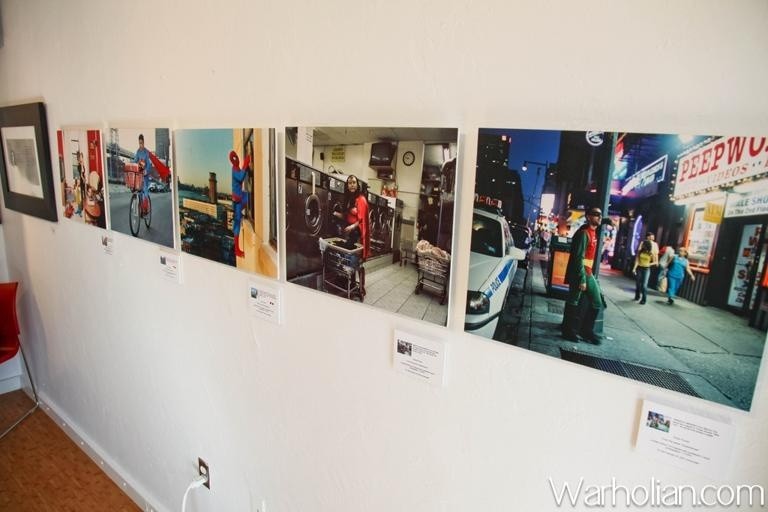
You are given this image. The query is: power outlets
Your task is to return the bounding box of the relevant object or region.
[195,459,210,490]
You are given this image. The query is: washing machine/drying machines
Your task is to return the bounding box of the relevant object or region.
[286,155,405,281]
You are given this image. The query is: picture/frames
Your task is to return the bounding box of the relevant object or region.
[0,101,57,222]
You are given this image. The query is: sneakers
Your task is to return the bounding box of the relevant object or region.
[634,296,675,305]
[561,333,601,345]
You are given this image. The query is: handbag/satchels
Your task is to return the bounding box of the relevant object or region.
[657,268,668,293]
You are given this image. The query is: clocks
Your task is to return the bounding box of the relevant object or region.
[403,152,415,166]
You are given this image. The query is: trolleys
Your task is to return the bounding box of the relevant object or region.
[400,240,450,305]
[319,236,366,303]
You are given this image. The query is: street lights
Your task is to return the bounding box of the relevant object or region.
[522,160,548,171]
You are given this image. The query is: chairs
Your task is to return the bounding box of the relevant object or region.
[0,282,38,439]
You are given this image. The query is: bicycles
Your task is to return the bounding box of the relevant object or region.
[124,163,152,237]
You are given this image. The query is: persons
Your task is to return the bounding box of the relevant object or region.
[632,240,656,304]
[76,151,86,205]
[332,175,368,297]
[639,232,659,268]
[132,134,151,216]
[562,206,603,345]
[606,237,614,264]
[228,150,251,257]
[538,227,555,254]
[665,247,696,305]
[657,244,675,292]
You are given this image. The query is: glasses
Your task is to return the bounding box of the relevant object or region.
[586,211,603,217]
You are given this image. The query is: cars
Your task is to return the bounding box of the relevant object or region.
[464,208,532,339]
